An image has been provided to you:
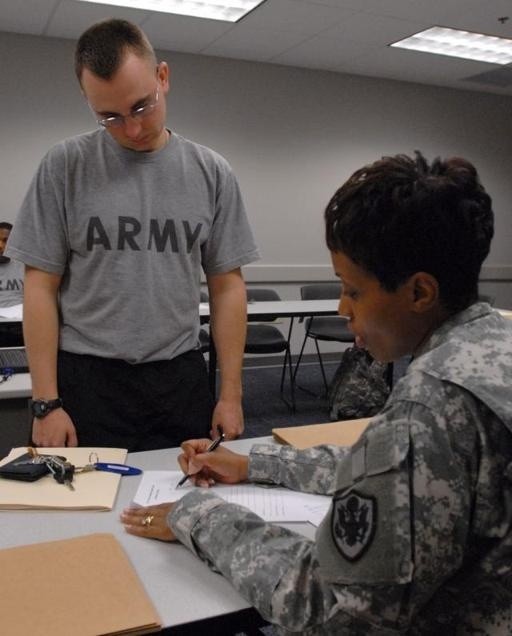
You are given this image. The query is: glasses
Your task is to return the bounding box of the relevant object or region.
[87,81,160,129]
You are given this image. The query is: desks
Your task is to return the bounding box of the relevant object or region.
[0,433,335,636]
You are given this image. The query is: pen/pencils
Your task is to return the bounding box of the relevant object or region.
[177,434,225,488]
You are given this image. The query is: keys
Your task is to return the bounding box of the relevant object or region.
[12,449,143,491]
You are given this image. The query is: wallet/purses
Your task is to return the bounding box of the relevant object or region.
[0,452,66,482]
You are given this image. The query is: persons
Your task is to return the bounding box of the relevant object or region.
[4,19,258,448]
[0,224,24,310]
[120,148,512,636]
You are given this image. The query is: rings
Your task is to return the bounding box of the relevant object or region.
[141,514,154,525]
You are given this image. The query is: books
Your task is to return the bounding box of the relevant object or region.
[0,447,128,510]
[0,534,162,636]
[271,418,369,448]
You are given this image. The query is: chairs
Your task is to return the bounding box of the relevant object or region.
[0,279,358,417]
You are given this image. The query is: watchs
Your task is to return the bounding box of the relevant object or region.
[29,398,63,418]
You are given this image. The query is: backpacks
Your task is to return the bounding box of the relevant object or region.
[324,345,395,422]
